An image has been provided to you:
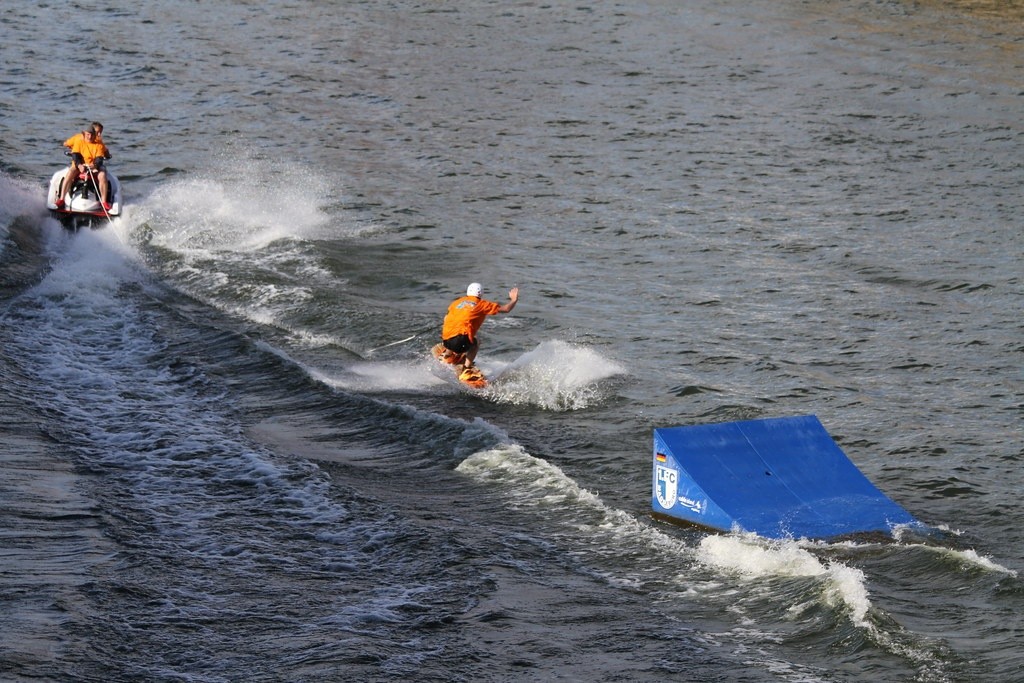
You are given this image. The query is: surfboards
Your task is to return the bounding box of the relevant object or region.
[432,342,485,389]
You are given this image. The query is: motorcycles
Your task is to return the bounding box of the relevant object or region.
[47,164,124,232]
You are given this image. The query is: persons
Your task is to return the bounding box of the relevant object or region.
[443,283,518,380]
[55,122,112,209]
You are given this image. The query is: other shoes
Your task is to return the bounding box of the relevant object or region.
[103,202,111,210]
[56,200,65,208]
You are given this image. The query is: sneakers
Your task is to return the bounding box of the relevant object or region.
[461,366,484,381]
[439,350,458,362]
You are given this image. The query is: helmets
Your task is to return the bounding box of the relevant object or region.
[466,283,484,299]
[86,126,96,133]
